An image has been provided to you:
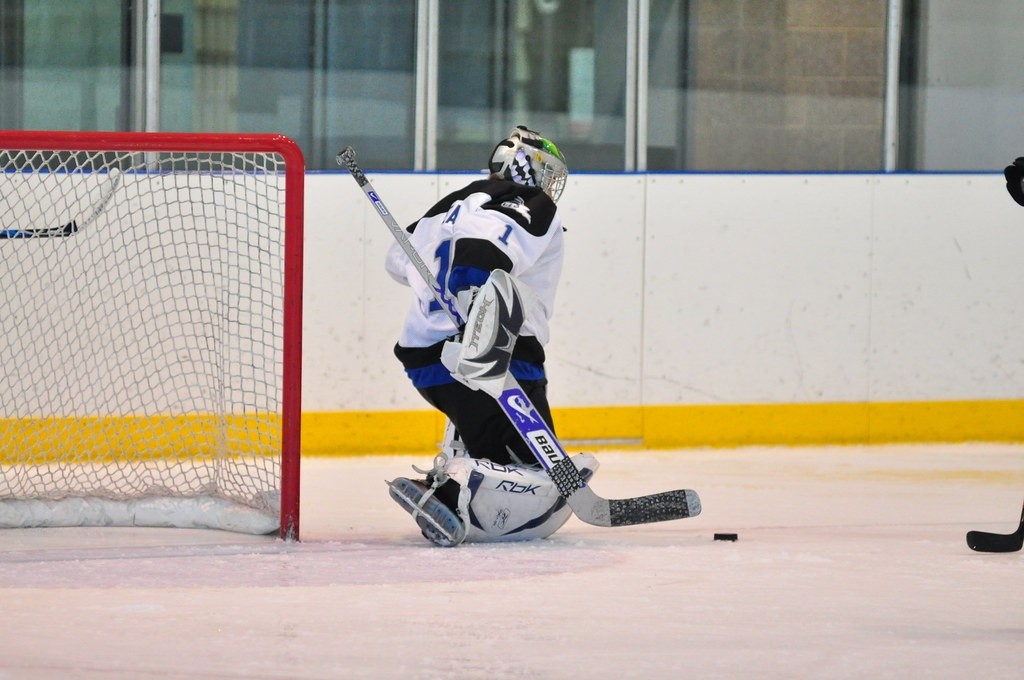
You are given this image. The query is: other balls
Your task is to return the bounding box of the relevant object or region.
[713,532,738,541]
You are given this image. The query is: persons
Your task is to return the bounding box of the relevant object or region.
[386,126,602,548]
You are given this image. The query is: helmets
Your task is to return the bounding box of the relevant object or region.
[488,124,568,205]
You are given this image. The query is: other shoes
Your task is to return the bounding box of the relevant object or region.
[384,475,465,548]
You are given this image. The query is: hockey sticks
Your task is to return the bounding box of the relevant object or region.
[0,163,122,243]
[965,506,1024,554]
[336,143,702,526]
